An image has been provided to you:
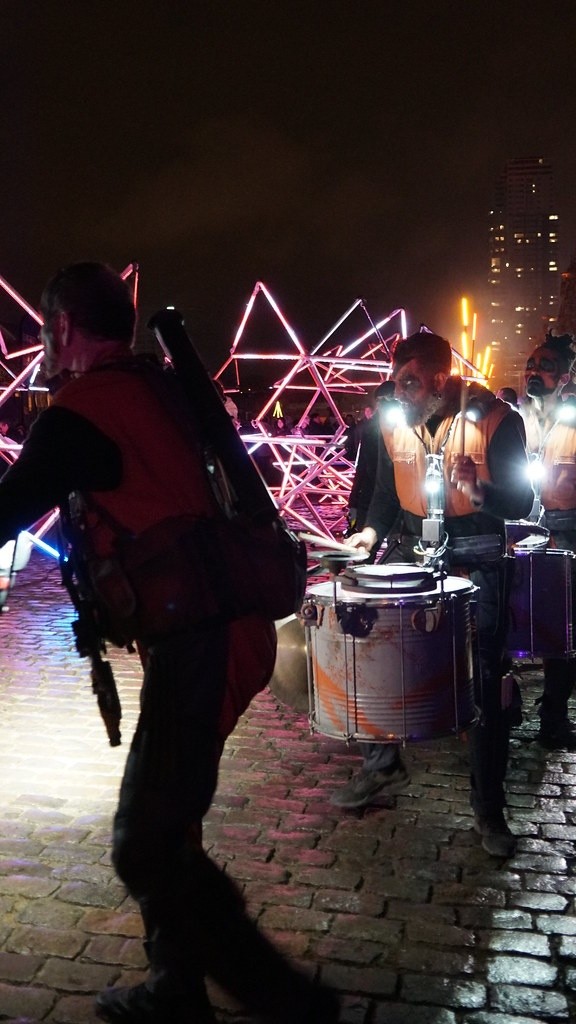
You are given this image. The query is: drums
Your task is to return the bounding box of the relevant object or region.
[501,546,576,662]
[305,562,480,750]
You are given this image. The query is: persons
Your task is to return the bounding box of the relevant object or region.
[330,328,576,857]
[239,406,376,484]
[0,263,342,1024]
[0,413,32,479]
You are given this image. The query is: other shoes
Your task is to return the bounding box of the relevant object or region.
[503,709,523,729]
[96,982,215,1024]
[534,694,576,744]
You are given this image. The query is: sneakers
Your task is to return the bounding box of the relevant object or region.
[330,759,411,807]
[474,812,515,857]
